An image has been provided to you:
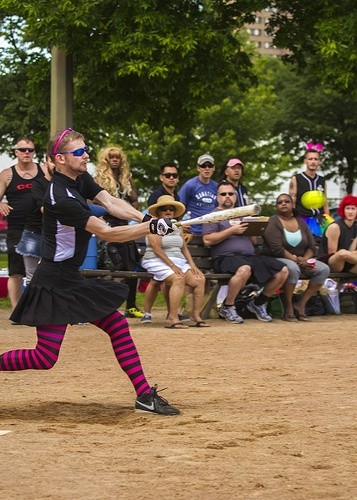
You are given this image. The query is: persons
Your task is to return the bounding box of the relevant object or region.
[320,196,357,278]
[200,181,290,325]
[287,141,331,241]
[212,158,253,309]
[1,128,183,417]
[140,159,191,325]
[140,195,212,330]
[15,154,58,296]
[0,138,54,327]
[263,193,331,324]
[172,155,224,321]
[87,142,149,319]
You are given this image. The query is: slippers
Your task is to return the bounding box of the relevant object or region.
[189,320,210,327]
[163,317,189,328]
[281,315,299,322]
[293,304,310,322]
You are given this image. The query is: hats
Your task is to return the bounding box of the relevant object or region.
[226,158,244,167]
[148,195,185,218]
[197,154,214,165]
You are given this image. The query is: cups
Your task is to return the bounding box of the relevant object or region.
[307,259,316,270]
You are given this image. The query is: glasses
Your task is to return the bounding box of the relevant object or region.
[55,146,88,156]
[198,163,215,168]
[17,148,36,153]
[277,200,291,205]
[161,173,179,179]
[217,192,236,196]
[156,206,176,212]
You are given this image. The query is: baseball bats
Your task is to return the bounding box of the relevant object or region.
[172,204,261,228]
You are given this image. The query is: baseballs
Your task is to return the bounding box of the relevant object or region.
[302,191,326,210]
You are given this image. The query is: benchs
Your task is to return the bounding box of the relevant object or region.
[83,222,357,323]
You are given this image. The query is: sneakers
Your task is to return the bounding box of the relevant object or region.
[218,303,243,324]
[135,384,181,416]
[124,307,146,318]
[140,312,152,324]
[247,299,272,322]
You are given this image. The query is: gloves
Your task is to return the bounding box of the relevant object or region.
[143,214,178,235]
